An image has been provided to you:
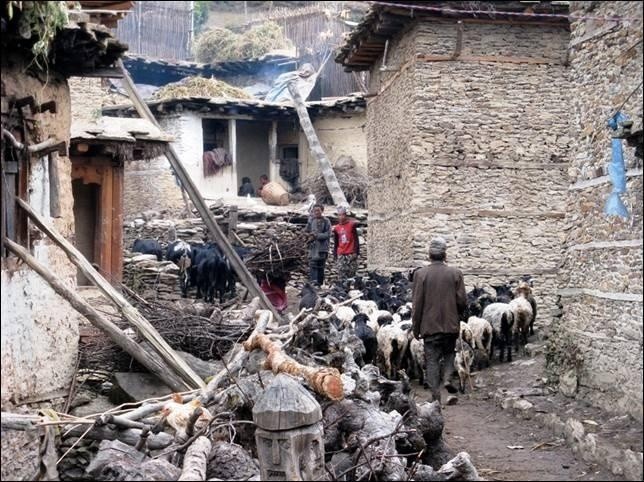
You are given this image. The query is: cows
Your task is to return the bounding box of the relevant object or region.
[162,238,252,304]
[131,236,163,261]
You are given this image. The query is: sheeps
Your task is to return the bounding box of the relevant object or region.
[295,267,537,395]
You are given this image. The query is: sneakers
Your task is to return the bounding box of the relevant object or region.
[444,380,458,394]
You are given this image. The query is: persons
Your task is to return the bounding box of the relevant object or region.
[411,237,468,404]
[333,205,359,282]
[256,174,269,197]
[238,177,254,196]
[304,204,331,286]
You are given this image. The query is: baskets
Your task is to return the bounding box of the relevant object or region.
[261,182,289,205]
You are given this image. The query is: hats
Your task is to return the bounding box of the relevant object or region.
[429,237,446,255]
